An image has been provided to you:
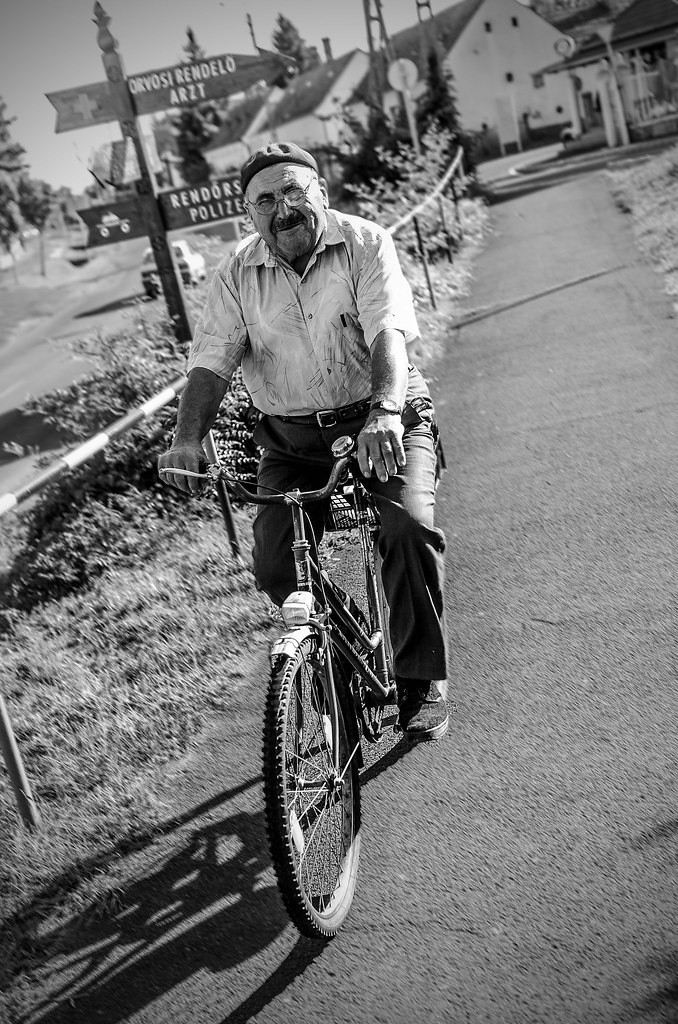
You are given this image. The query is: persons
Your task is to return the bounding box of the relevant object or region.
[158,140,451,741]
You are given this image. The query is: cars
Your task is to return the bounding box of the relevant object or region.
[139,240,207,300]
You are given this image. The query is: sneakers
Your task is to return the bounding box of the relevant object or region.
[311,594,359,715]
[395,674,448,742]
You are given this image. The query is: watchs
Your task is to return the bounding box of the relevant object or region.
[369,399,403,416]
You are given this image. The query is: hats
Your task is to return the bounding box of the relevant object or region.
[239,141,319,195]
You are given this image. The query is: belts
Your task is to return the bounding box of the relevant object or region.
[274,396,373,428]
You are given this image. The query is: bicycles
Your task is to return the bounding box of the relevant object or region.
[157,436,393,943]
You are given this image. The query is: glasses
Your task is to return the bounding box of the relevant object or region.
[247,177,313,215]
